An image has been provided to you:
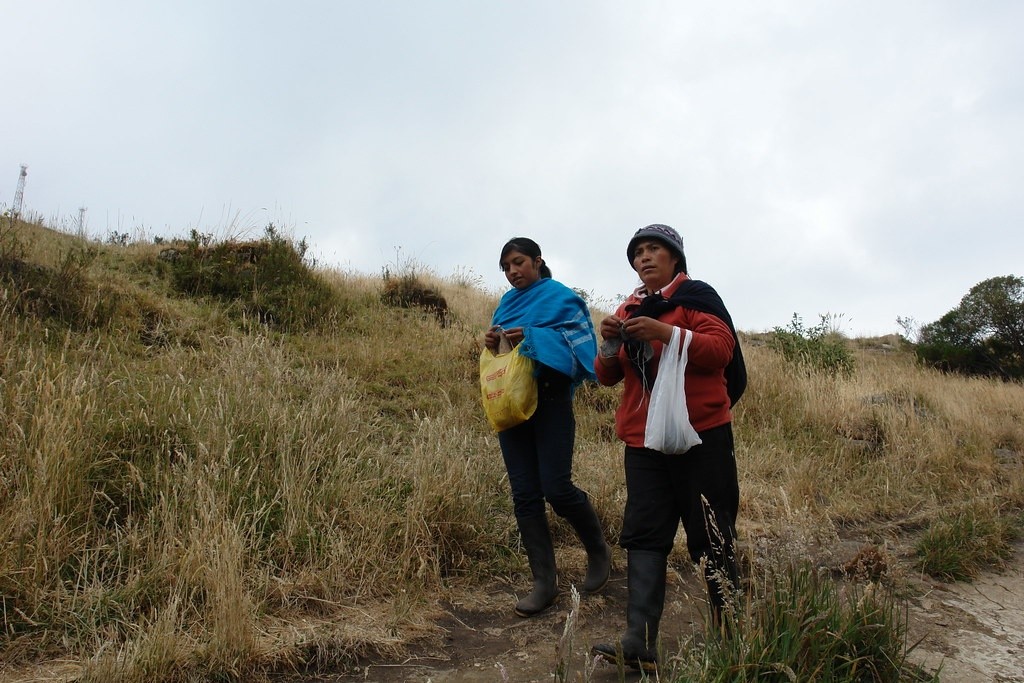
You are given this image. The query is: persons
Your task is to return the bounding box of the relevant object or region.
[481,237,613,617]
[590,223,741,674]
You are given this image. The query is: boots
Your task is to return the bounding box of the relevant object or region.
[689,535,742,644]
[565,495,613,596]
[512,538,560,618]
[590,546,666,674]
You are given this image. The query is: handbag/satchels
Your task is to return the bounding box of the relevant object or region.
[643,326,702,455]
[478,333,539,434]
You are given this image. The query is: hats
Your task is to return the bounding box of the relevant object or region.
[626,224,687,276]
